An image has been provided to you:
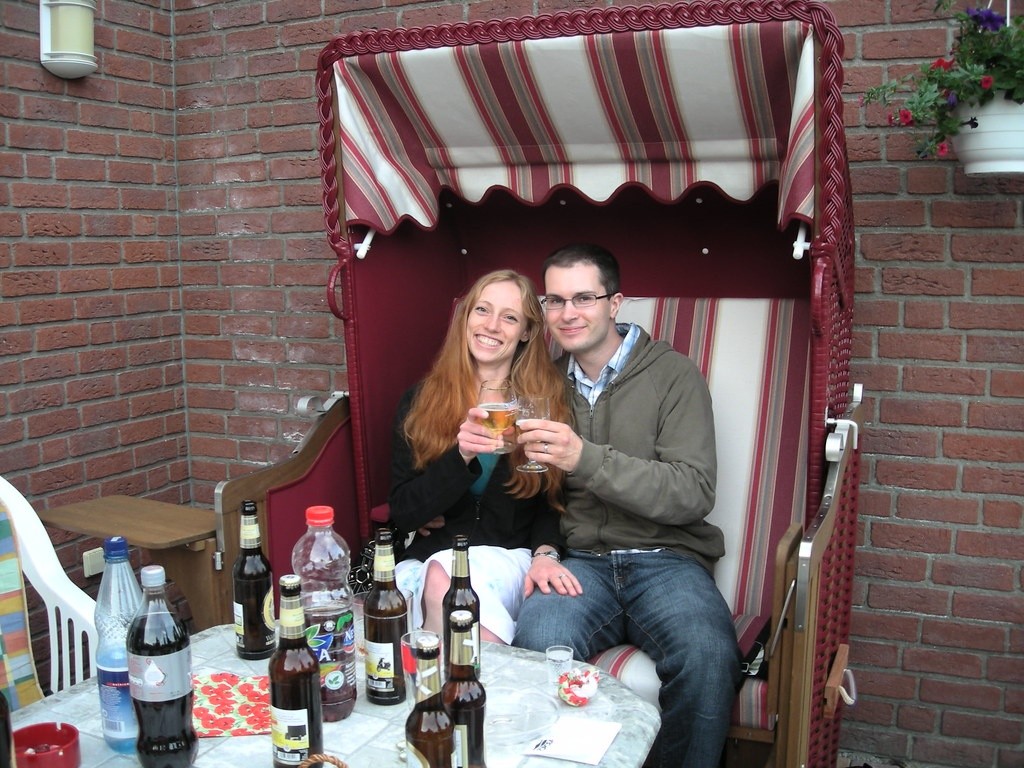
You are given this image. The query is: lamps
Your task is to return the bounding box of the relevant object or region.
[38,0,98,78]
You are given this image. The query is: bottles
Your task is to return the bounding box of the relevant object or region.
[269,575,324,768]
[406,637,457,768]
[442,533,482,682]
[127,565,199,768]
[0,689,13,767]
[363,527,407,705]
[231,499,276,660]
[93,535,142,754]
[291,506,357,723]
[442,610,487,768]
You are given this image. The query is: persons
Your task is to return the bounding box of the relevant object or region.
[389,270,582,645]
[510,244,742,768]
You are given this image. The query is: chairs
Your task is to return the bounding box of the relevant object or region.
[0,475,100,716]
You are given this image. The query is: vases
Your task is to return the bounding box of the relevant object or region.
[949,88,1024,177]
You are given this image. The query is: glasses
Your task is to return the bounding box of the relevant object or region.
[541,293,608,308]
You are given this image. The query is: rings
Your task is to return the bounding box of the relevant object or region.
[544,443,549,453]
[560,573,568,578]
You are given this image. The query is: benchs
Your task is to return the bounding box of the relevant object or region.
[366,290,808,730]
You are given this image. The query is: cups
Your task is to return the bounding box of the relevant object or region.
[516,395,551,473]
[545,645,574,698]
[478,380,517,453]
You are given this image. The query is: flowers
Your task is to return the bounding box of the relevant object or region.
[863,0,1024,160]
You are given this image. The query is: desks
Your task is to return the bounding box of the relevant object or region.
[35,496,224,634]
[9,622,662,768]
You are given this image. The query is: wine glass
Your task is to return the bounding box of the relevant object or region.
[395,630,442,761]
